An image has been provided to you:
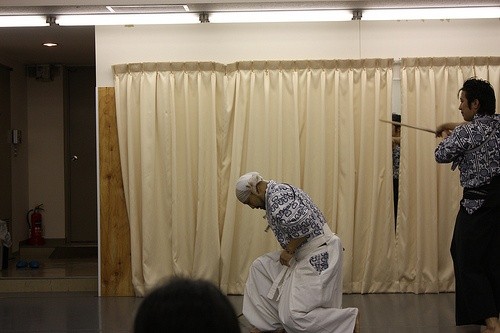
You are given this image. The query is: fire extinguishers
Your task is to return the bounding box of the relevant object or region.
[27,203,44,244]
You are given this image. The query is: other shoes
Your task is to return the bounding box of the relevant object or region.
[16,261,24,267]
[30,261,38,267]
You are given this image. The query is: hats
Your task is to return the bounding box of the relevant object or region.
[235,172,263,203]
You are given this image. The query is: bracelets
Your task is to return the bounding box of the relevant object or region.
[285,248,293,255]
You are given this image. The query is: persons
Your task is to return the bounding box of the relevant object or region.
[133,277,241,333]
[236,171,361,333]
[434,79,500,333]
